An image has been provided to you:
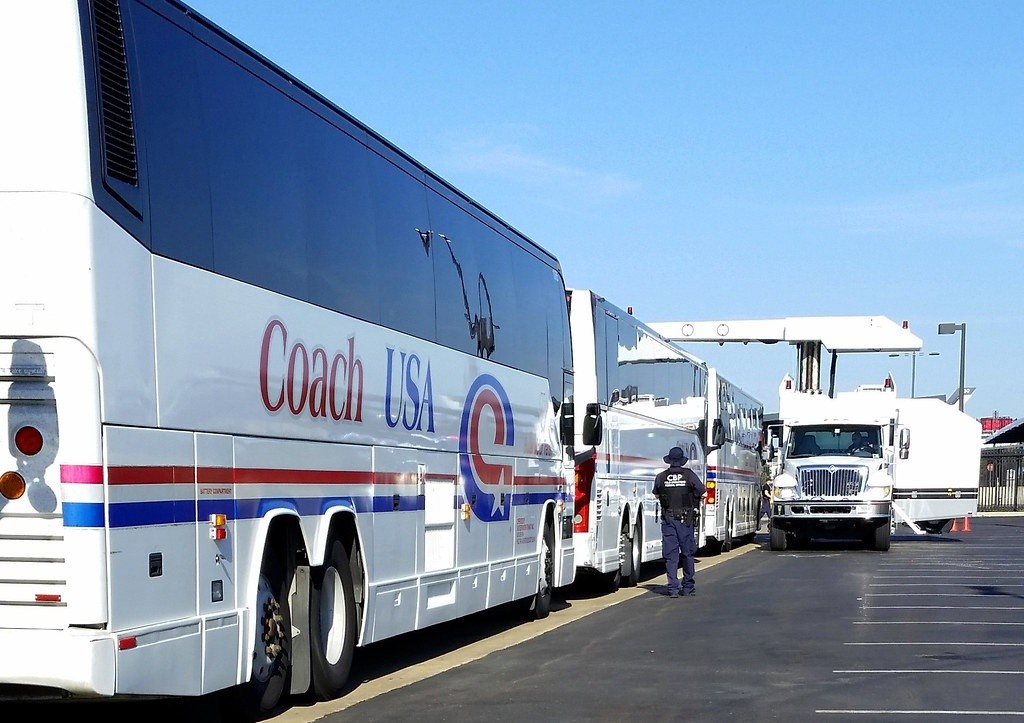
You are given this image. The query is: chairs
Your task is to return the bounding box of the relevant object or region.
[861,436,869,446]
[795,435,822,454]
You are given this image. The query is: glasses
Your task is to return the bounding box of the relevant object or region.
[852,437,858,441]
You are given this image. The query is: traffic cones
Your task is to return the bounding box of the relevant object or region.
[962,516,972,531]
[950,519,958,532]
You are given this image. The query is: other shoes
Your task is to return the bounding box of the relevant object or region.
[682,588,696,596]
[670,592,680,598]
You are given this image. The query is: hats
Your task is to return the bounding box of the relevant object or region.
[663,447,688,465]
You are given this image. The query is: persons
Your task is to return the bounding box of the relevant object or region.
[759,478,773,519]
[847,432,868,450]
[652,447,706,598]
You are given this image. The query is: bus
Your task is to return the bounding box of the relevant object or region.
[566,287,725,594]
[0,0,602,723]
[705,368,764,551]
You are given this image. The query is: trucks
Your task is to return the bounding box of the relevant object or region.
[768,374,982,551]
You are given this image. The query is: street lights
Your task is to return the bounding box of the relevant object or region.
[938,323,965,413]
[889,352,940,398]
